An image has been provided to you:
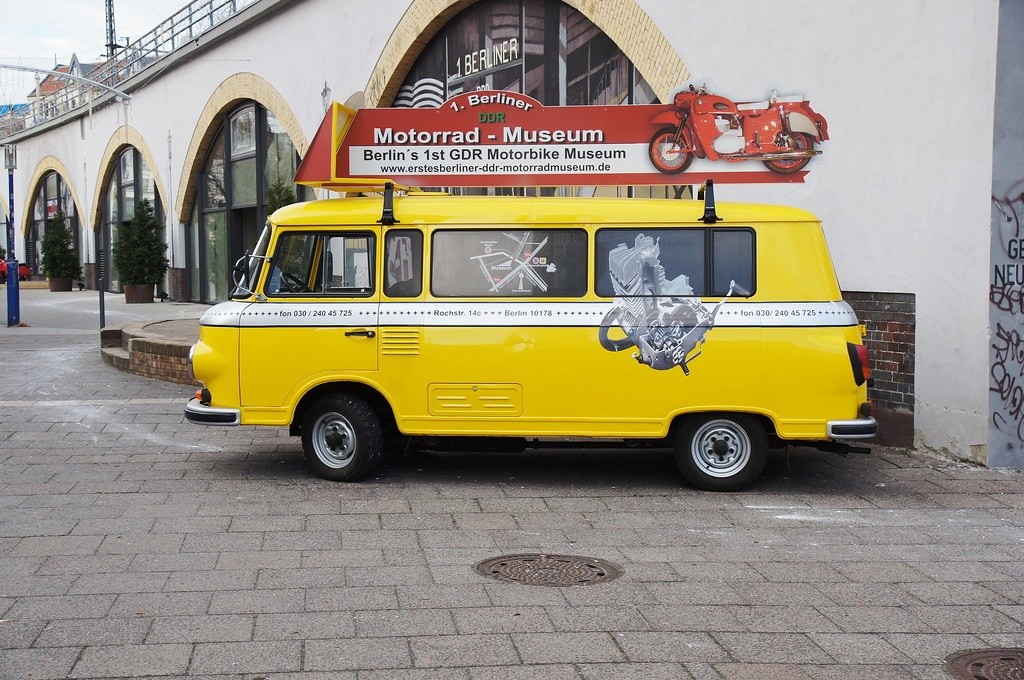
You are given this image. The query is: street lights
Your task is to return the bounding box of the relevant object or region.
[3,143,21,327]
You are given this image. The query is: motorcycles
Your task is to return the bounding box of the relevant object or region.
[645,83,830,176]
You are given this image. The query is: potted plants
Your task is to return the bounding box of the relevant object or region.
[107,198,169,304]
[39,209,79,293]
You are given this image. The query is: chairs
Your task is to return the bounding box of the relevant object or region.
[384,279,424,297]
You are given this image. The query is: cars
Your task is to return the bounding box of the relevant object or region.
[0,259,33,284]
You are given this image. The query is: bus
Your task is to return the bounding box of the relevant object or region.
[182,192,876,492]
